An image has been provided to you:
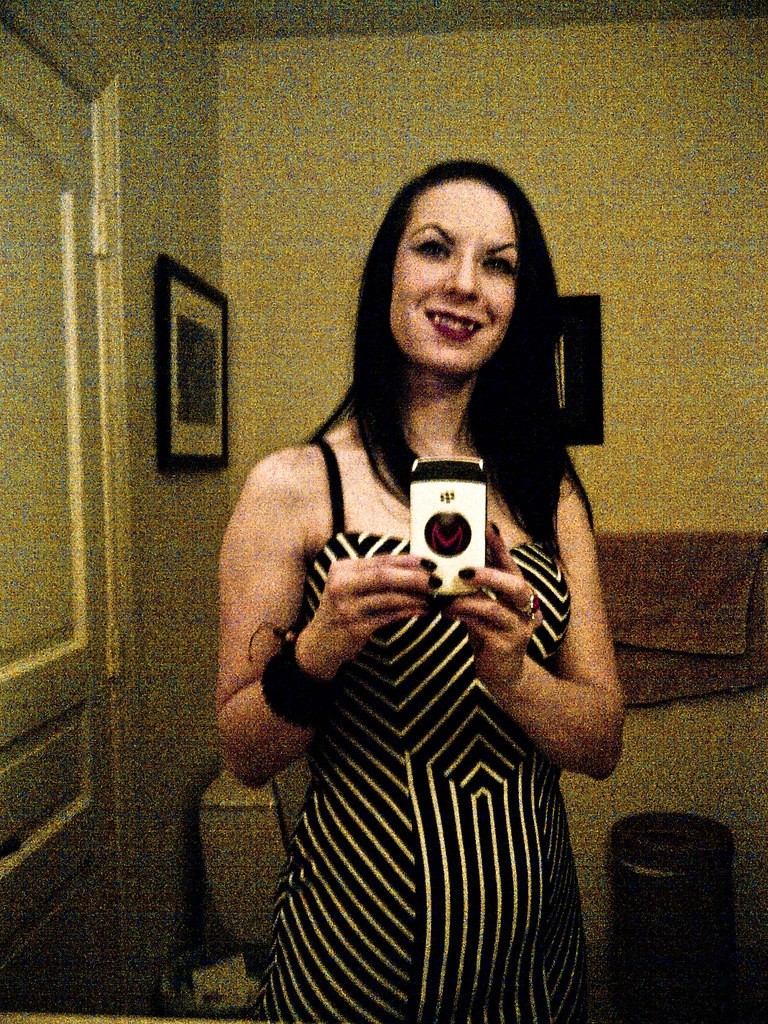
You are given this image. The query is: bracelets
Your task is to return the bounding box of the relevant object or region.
[247,621,337,728]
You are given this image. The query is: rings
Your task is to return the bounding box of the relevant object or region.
[518,586,541,619]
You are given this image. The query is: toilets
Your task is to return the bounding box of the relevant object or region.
[196,753,314,955]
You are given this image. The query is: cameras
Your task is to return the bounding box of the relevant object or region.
[408,456,488,597]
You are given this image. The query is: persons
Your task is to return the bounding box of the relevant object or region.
[213,159,627,1024]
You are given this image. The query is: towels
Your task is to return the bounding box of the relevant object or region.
[594,531,768,706]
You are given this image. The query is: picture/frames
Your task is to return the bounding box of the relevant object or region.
[554,291,607,447]
[150,251,229,476]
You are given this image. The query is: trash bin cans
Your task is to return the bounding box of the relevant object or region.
[605,811,734,1024]
